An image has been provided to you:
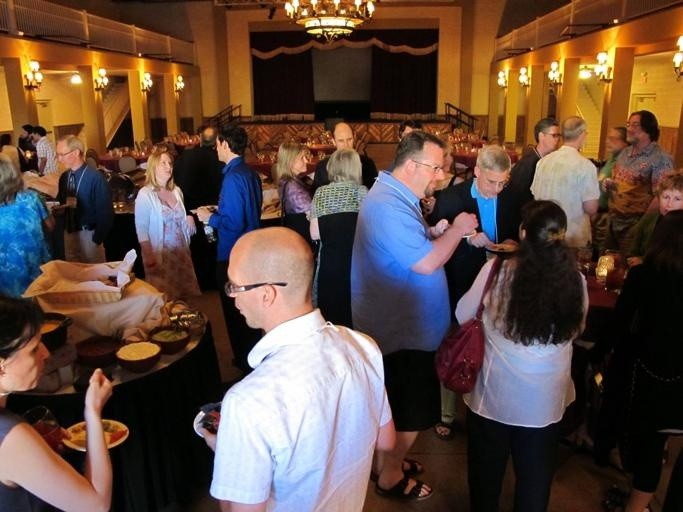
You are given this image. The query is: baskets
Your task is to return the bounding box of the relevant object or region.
[38,262,124,303]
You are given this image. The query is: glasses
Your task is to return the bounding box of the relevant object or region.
[548,133,561,137]
[413,158,440,173]
[224,280,288,297]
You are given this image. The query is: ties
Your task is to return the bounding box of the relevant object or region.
[64,177,76,230]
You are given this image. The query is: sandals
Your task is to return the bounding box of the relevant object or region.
[376,476,435,503]
[435,417,458,440]
[369,457,424,481]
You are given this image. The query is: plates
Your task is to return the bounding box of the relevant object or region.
[486,243,518,254]
[63,419,130,452]
[192,401,225,438]
[462,229,477,239]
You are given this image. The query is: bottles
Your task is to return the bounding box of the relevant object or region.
[203,219,217,243]
[108,146,144,157]
[163,133,198,145]
[21,404,62,449]
[577,245,625,291]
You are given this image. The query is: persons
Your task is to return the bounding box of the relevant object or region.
[599,210,683,511]
[197,225,399,512]
[347,129,479,503]
[0,297,116,511]
[453,199,589,512]
[1,109,683,449]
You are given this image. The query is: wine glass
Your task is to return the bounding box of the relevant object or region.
[432,129,478,155]
[256,150,277,163]
[307,130,335,163]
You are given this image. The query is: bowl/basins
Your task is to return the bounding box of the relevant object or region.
[39,311,75,351]
[115,326,192,373]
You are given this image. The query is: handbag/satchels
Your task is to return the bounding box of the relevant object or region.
[433,319,484,394]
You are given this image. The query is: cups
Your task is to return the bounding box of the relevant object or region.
[606,178,613,192]
[25,150,31,159]
[113,201,125,212]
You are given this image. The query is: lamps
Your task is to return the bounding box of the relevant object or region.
[672,35,682,79]
[23,61,43,90]
[279,0,379,46]
[93,69,184,94]
[497,52,612,89]
